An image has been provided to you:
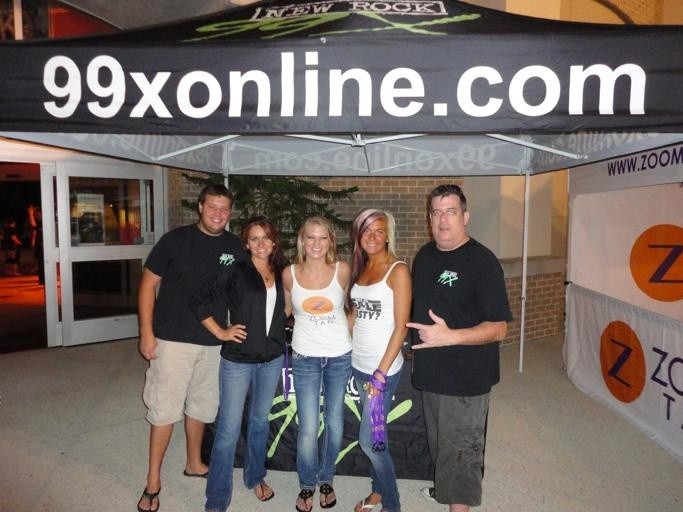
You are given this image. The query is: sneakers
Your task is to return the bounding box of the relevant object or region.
[421,487,436,499]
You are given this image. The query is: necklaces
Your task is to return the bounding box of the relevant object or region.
[256,270,275,285]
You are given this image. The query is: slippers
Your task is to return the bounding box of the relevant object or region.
[137,470,381,512]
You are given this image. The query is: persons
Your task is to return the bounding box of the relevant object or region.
[343,207,412,512]
[282,213,353,512]
[134,182,246,512]
[403,182,516,512]
[188,214,285,512]
